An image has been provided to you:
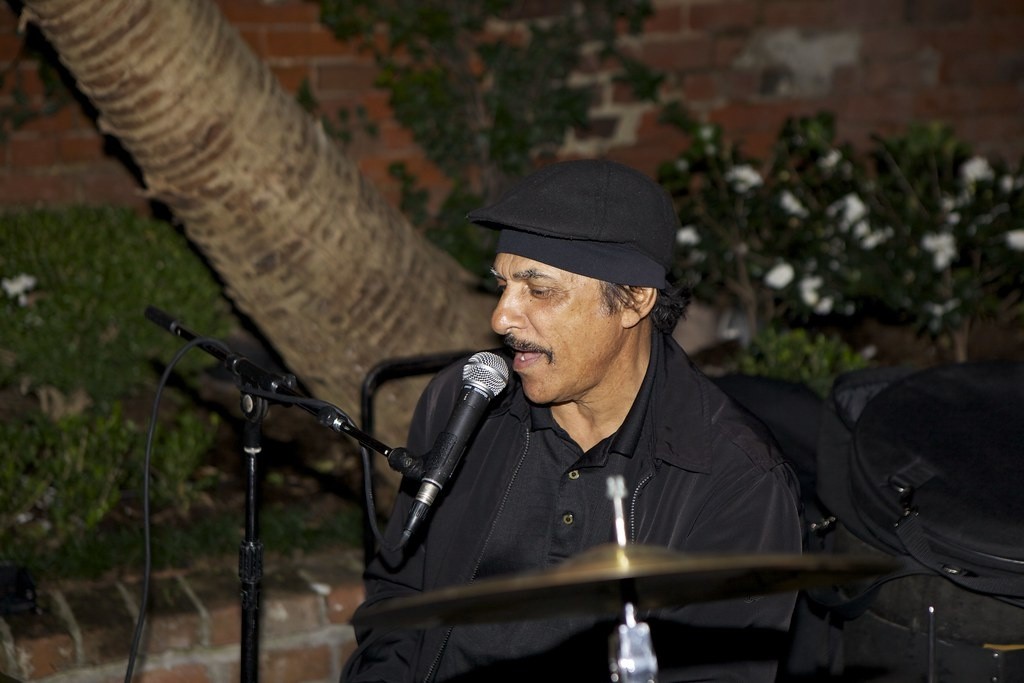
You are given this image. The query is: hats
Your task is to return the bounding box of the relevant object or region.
[467,160,679,289]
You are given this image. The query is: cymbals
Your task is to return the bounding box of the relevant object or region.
[335,539,913,636]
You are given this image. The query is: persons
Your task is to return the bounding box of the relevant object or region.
[343,159,802,683]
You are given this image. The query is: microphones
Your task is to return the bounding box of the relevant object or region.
[396,352,511,546]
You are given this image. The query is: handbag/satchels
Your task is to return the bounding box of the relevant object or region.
[848,359,1024,602]
